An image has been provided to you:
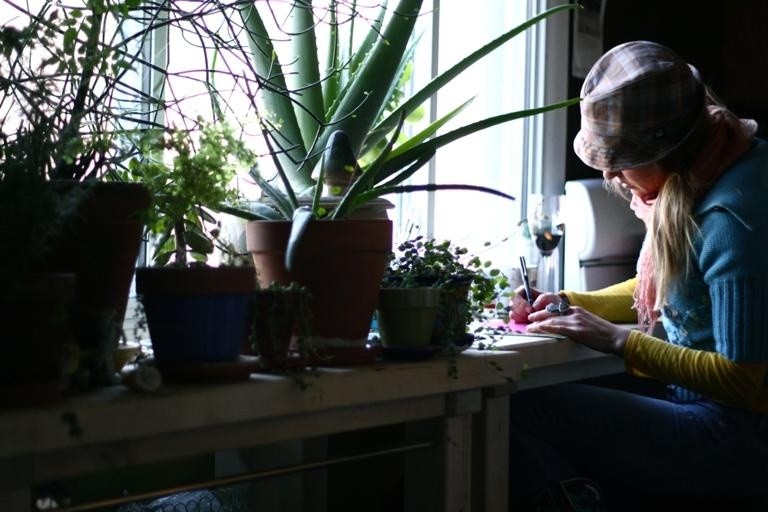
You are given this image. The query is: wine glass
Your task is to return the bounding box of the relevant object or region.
[526,193,567,292]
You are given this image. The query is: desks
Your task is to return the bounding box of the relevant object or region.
[1,316,670,512]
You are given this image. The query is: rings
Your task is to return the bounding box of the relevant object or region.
[546,298,569,315]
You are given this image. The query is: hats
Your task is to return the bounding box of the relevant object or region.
[573,40,705,173]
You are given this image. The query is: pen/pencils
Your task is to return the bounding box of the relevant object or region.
[519,256,534,306]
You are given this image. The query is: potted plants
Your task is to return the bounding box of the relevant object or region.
[385,236,512,345]
[0,0,387,407]
[225,0,583,360]
[379,269,442,357]
[116,127,262,379]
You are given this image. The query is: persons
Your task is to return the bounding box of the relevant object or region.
[506,42,767,510]
